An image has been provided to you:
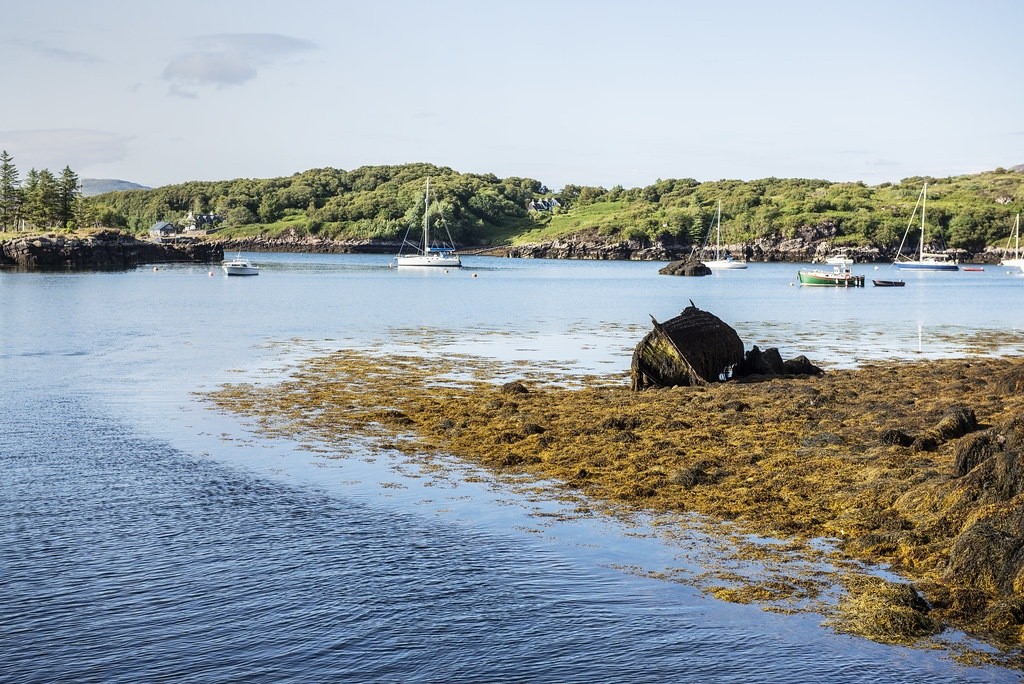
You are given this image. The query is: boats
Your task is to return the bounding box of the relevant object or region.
[636,299,744,384]
[872,279,905,288]
[826,254,854,265]
[798,259,865,287]
[962,267,984,271]
[222,247,260,277]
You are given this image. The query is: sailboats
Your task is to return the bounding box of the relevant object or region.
[394,175,462,267]
[1000,212,1024,266]
[892,181,959,271]
[700,199,749,269]
